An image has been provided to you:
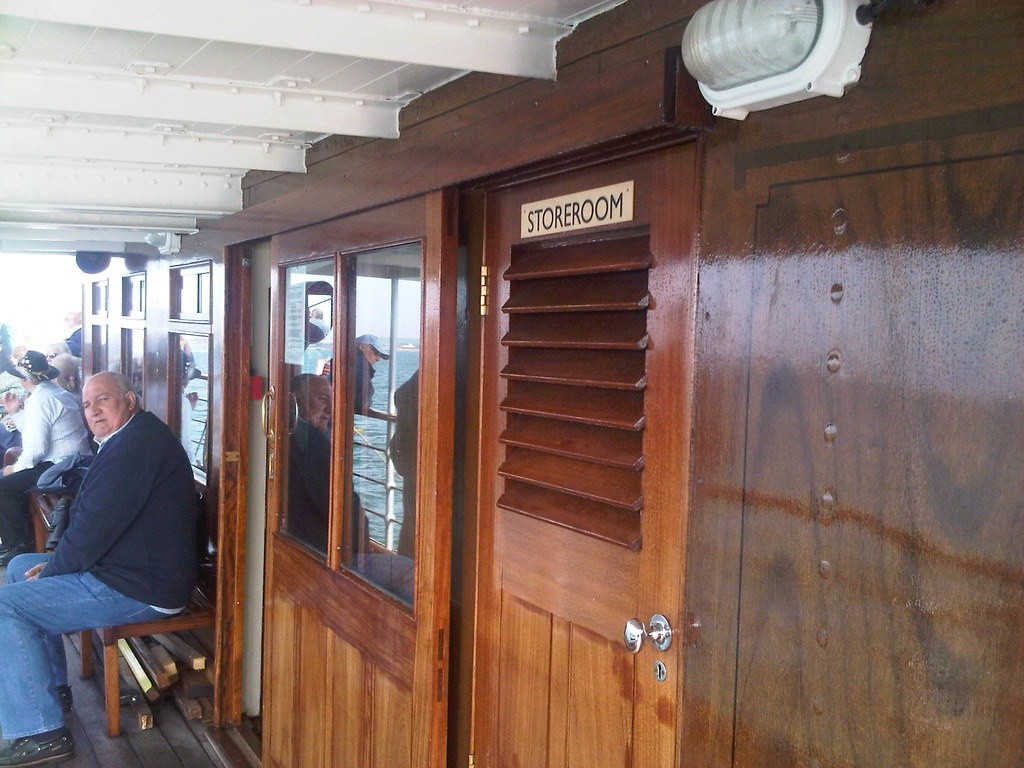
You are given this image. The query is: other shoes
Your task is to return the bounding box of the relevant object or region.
[0,541,35,566]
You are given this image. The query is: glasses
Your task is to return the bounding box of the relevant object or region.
[43,353,58,359]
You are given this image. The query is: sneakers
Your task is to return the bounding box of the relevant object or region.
[0,729,76,768]
[58,685,73,713]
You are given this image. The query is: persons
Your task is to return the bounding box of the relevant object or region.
[136,334,198,466]
[285,373,372,559]
[389,369,419,561]
[319,335,390,440]
[0,309,91,568]
[0,371,201,768]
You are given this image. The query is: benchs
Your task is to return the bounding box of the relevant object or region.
[30,478,218,738]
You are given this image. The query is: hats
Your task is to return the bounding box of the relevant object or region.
[356,334,391,361]
[6,351,60,382]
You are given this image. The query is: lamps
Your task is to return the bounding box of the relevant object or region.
[680,0,890,121]
